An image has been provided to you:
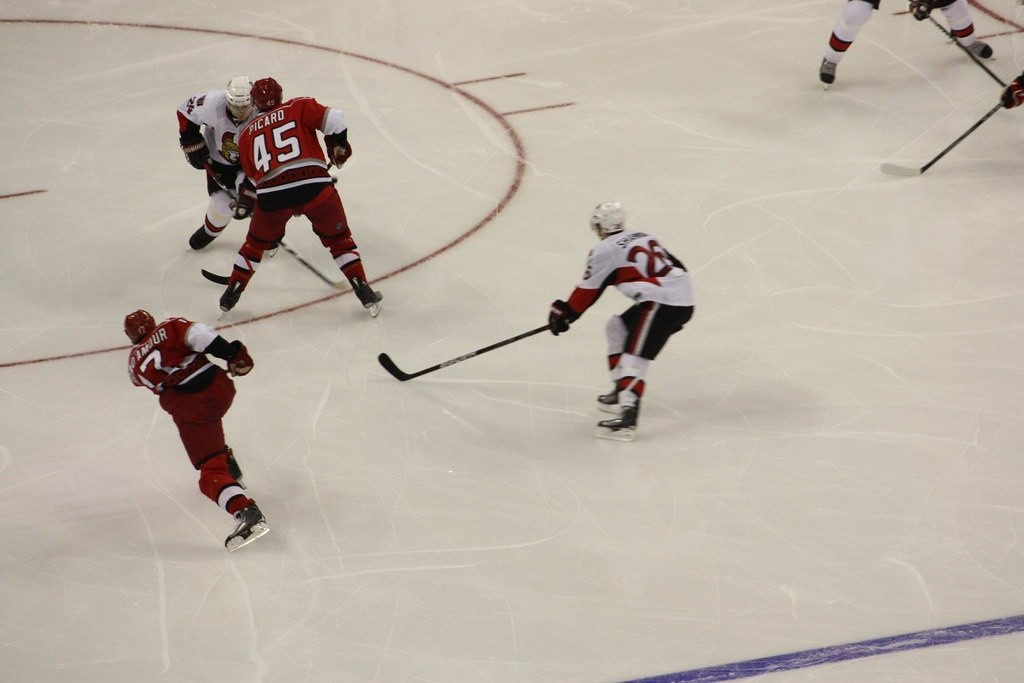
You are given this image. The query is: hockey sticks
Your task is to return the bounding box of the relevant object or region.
[203,163,353,289]
[200,158,334,284]
[879,101,1004,178]
[378,324,550,381]
[910,0,1007,87]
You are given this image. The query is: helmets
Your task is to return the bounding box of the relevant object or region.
[591,202,624,234]
[250,78,283,113]
[124,310,156,344]
[226,76,253,108]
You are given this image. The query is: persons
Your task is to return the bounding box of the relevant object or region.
[549,202,694,430]
[124,309,264,546]
[818,1,993,83]
[1000,71,1024,110]
[176,75,261,249]
[221,77,383,312]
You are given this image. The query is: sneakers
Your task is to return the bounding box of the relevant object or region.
[598,391,621,414]
[225,446,247,489]
[217,280,242,321]
[820,56,838,90]
[967,41,995,60]
[596,419,637,441]
[225,504,270,552]
[188,224,217,251]
[352,276,382,318]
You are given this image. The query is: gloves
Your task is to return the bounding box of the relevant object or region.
[910,0,931,21]
[227,340,253,377]
[549,300,579,336]
[179,133,212,170]
[324,128,352,167]
[1002,78,1024,109]
[232,188,256,220]
